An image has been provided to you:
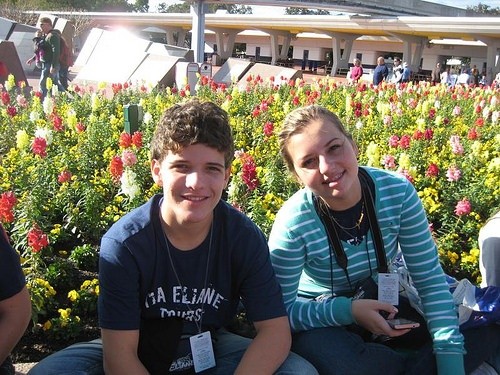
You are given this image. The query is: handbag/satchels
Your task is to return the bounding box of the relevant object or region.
[393,252,500,375]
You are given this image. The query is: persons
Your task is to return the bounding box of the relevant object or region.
[26,17,68,101]
[430,61,500,91]
[27,97,321,375]
[349,58,363,85]
[0,218,32,375]
[373,56,390,87]
[400,61,412,84]
[52,27,72,92]
[477,209,500,303]
[390,56,407,85]
[32,30,47,68]
[257,105,500,375]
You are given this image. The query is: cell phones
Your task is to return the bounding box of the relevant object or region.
[384,316,421,329]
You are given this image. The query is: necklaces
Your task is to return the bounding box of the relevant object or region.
[326,198,367,232]
